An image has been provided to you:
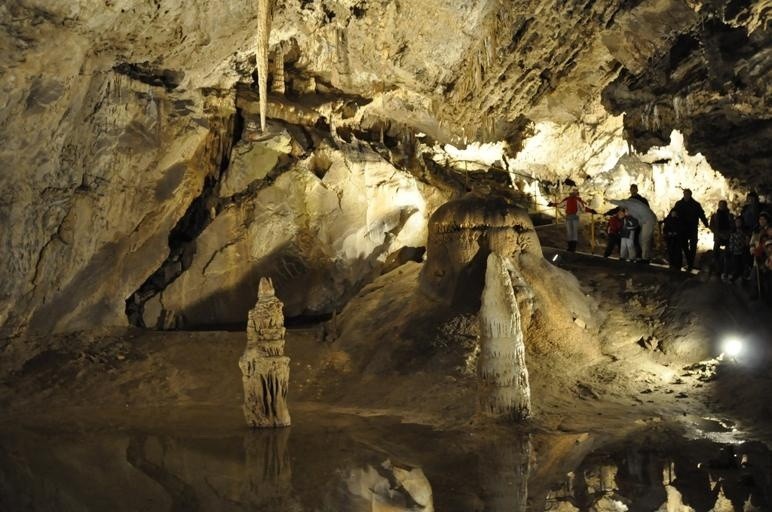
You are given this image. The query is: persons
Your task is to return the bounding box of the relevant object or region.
[602,183,772,288]
[547,190,598,252]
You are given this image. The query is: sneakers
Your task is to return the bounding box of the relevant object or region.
[602,253,751,284]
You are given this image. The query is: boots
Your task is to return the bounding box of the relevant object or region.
[565,239,578,253]
